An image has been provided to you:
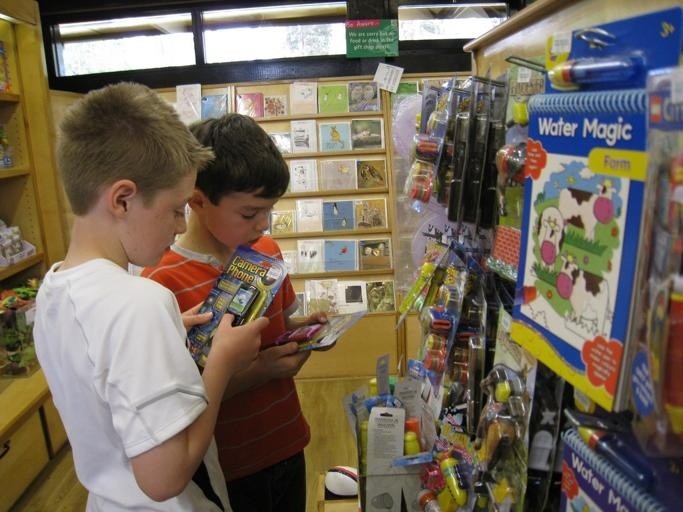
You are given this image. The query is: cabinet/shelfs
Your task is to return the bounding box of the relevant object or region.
[231,72,404,384]
[458,0,681,107]
[0,1,71,511]
[385,69,473,377]
[125,83,232,275]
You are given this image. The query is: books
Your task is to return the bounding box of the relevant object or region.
[509,89,660,412]
[563,430,669,512]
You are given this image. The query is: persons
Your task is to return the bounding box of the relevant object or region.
[139,113,336,512]
[33,83,270,511]
